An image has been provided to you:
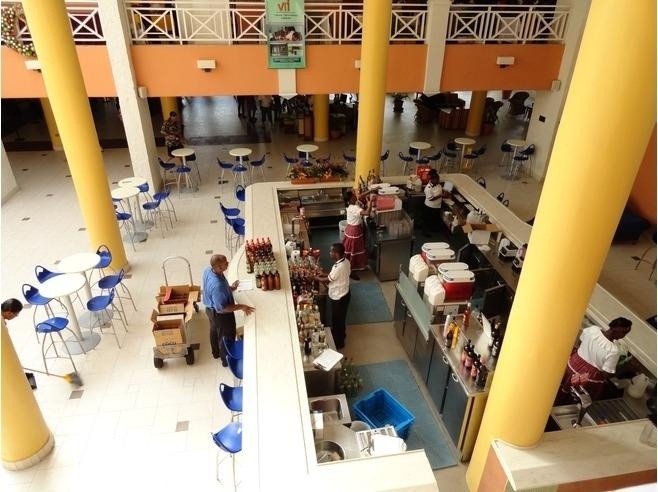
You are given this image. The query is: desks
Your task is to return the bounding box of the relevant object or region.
[39,275,100,355]
[296,144,319,162]
[170,148,195,184]
[119,177,154,229]
[111,187,148,243]
[409,141,431,173]
[506,139,526,180]
[229,147,253,186]
[455,138,476,172]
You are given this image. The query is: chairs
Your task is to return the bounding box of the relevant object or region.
[513,153,529,182]
[229,222,245,256]
[112,197,128,223]
[35,317,87,372]
[157,157,181,194]
[302,161,313,168]
[88,290,130,351]
[408,146,417,159]
[442,145,460,172]
[472,144,487,171]
[518,143,536,177]
[282,152,299,177]
[143,196,168,238]
[173,164,196,199]
[316,154,331,170]
[114,209,138,251]
[19,283,68,331]
[298,150,313,160]
[398,151,414,175]
[219,382,243,419]
[225,354,243,379]
[342,152,355,170]
[379,150,389,176]
[633,228,656,280]
[446,138,460,172]
[225,216,245,258]
[184,154,201,179]
[462,153,477,174]
[152,188,177,227]
[428,150,441,175]
[219,202,241,246]
[34,264,86,311]
[232,165,247,189]
[215,156,235,184]
[234,184,245,209]
[249,154,266,179]
[84,244,121,297]
[415,155,429,173]
[136,184,154,216]
[222,335,243,357]
[212,420,241,468]
[500,140,515,169]
[235,156,249,173]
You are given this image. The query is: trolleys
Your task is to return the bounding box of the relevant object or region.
[153,256,199,369]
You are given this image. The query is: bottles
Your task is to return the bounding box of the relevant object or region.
[460,338,472,362]
[445,313,462,348]
[487,321,501,347]
[296,304,328,356]
[461,302,473,327]
[464,345,476,369]
[475,363,487,386]
[491,334,502,357]
[288,249,320,296]
[470,354,483,378]
[243,236,281,291]
[386,215,411,240]
[340,356,363,394]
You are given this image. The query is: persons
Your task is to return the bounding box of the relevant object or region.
[341,186,382,280]
[420,169,451,238]
[201,254,256,368]
[558,317,632,406]
[233,95,282,124]
[161,112,183,157]
[305,243,351,350]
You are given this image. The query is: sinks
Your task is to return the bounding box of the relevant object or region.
[311,398,344,422]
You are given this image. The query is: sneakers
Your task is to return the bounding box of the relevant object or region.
[350,271,360,280]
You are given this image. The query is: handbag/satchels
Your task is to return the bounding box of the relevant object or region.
[369,434,405,455]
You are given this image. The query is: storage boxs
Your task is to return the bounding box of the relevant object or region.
[461,222,499,244]
[156,284,197,325]
[149,305,190,338]
[153,319,188,353]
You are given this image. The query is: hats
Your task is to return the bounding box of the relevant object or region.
[343,192,353,201]
[609,317,632,326]
